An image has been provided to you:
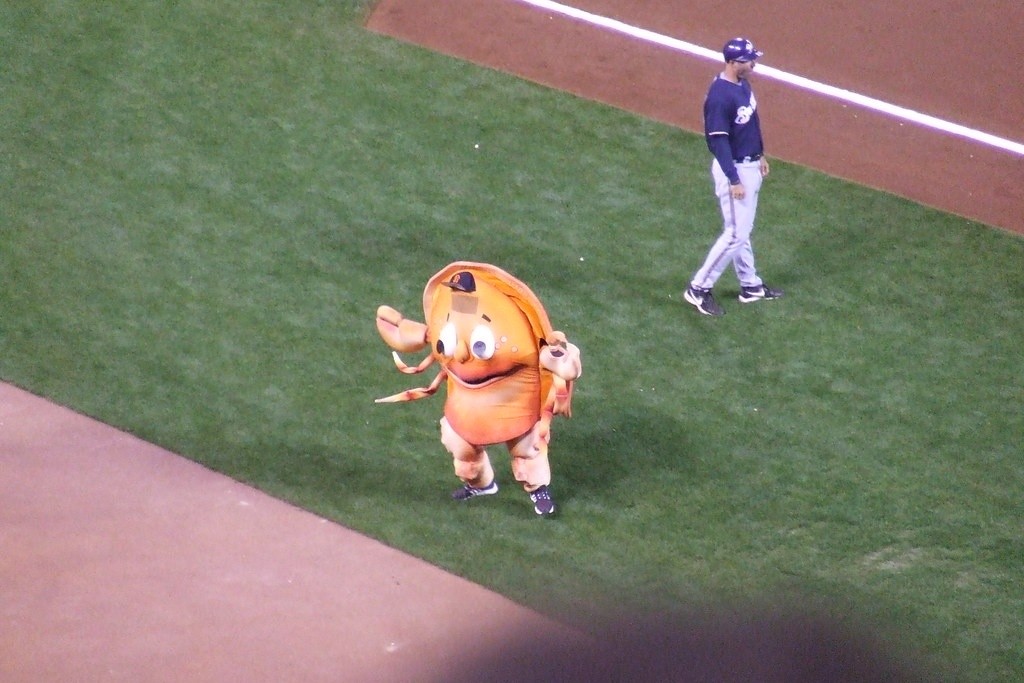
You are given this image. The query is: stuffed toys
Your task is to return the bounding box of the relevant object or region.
[374,260,583,515]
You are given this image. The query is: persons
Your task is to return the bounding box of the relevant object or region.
[683,37,784,315]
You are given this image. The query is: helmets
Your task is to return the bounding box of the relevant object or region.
[723,38,764,62]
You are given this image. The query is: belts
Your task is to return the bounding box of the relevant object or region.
[736,152,763,163]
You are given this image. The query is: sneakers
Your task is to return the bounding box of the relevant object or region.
[739,284,784,303]
[684,286,726,316]
